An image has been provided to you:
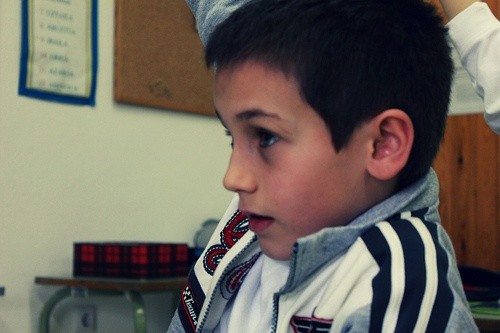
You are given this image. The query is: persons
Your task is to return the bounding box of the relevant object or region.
[165,1,479,333]
[437,1,500,136]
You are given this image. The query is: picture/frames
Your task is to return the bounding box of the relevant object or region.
[18,0,98,106]
[115,1,215,116]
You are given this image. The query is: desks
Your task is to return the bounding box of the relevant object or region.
[34,276,187,333]
